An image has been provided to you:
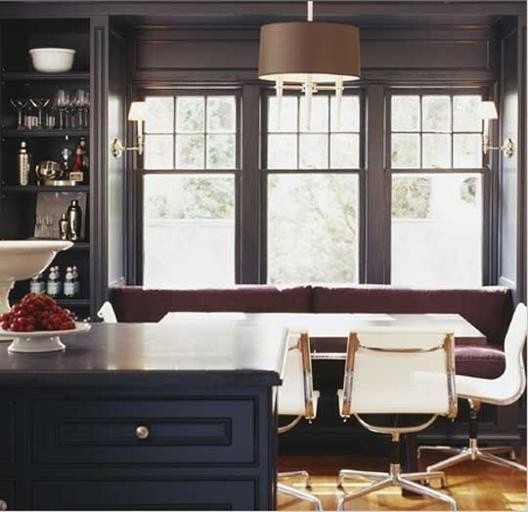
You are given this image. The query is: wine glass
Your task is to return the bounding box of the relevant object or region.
[34,212,59,239]
[7,87,90,132]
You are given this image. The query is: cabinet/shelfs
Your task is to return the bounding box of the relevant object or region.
[0,0,129,321]
[0,321,294,511]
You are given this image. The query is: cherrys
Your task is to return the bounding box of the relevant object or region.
[0,292,75,331]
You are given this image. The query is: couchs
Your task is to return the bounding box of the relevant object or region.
[108,285,516,379]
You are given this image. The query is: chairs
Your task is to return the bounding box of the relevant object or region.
[98,301,118,324]
[416,302,527,486]
[337,327,460,512]
[277,329,323,510]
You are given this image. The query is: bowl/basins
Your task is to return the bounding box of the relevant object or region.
[28,47,77,73]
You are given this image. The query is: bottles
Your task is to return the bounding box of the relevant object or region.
[59,212,70,241]
[16,140,31,187]
[29,266,81,298]
[71,143,82,172]
[67,200,82,242]
[79,136,90,185]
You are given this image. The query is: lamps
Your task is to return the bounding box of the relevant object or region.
[113,101,150,159]
[258,3,360,128]
[477,101,512,157]
